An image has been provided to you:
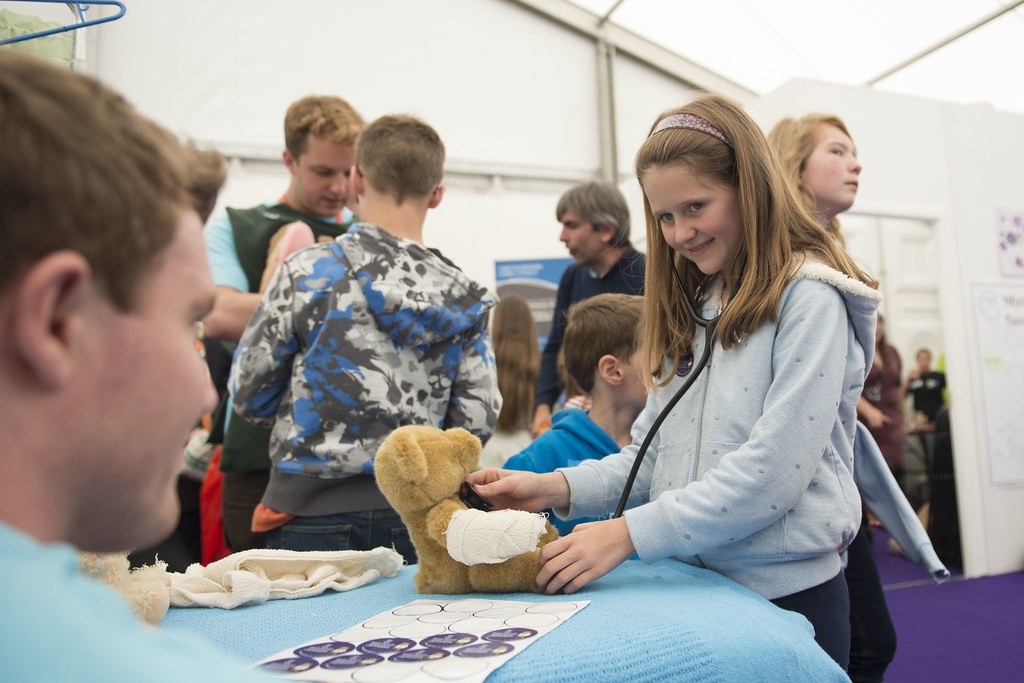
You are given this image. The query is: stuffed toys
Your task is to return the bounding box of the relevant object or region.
[372,425,564,593]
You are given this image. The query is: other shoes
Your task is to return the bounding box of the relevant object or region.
[868,518,881,526]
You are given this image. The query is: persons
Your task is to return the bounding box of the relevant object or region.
[228,114,504,556]
[766,115,947,573]
[479,181,646,561]
[0,51,290,683]
[462,99,883,676]
[127,94,371,570]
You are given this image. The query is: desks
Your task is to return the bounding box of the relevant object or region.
[170,555,856,683]
